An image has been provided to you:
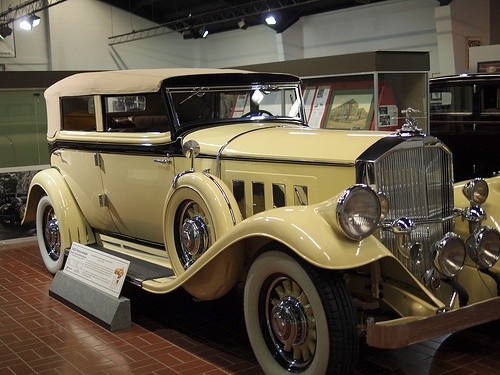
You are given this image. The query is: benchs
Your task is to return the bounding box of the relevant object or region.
[107,114,169,131]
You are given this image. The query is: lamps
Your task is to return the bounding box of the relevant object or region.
[182,27,192,39]
[28,13,41,28]
[0,24,12,38]
[198,26,208,38]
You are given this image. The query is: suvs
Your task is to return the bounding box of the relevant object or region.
[20,66,500,375]
[429,72,500,185]
